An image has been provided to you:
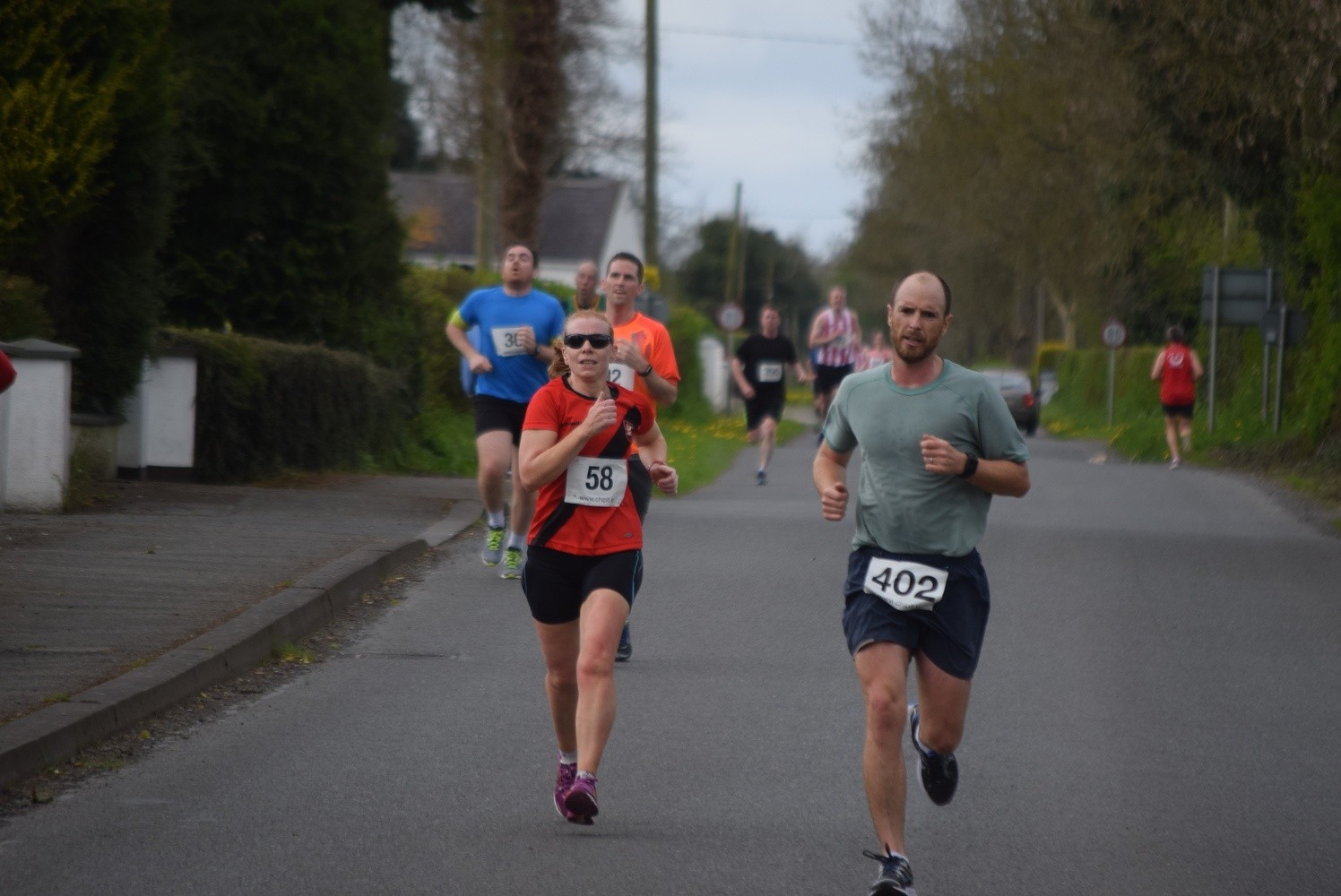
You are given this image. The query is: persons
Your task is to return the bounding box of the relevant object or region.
[563,250,683,660]
[808,269,1031,895]
[519,306,680,827]
[1150,322,1202,470]
[729,303,818,485]
[443,244,566,581]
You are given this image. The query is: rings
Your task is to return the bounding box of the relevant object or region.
[929,455,934,465]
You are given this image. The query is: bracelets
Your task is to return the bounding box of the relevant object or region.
[646,459,666,480]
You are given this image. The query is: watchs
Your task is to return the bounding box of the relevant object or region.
[637,366,652,377]
[957,449,978,481]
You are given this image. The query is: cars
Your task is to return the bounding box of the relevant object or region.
[970,369,1040,435]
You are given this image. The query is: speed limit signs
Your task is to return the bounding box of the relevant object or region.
[1102,322,1128,349]
[719,305,744,331]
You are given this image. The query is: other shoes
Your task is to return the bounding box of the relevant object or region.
[756,471,766,485]
[1175,460,1179,477]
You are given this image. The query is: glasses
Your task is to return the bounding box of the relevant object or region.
[562,334,612,349]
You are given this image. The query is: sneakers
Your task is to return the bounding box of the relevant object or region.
[565,777,598,826]
[908,703,958,805]
[862,843,915,896]
[500,549,524,578]
[554,755,585,817]
[481,525,506,566]
[615,621,631,660]
